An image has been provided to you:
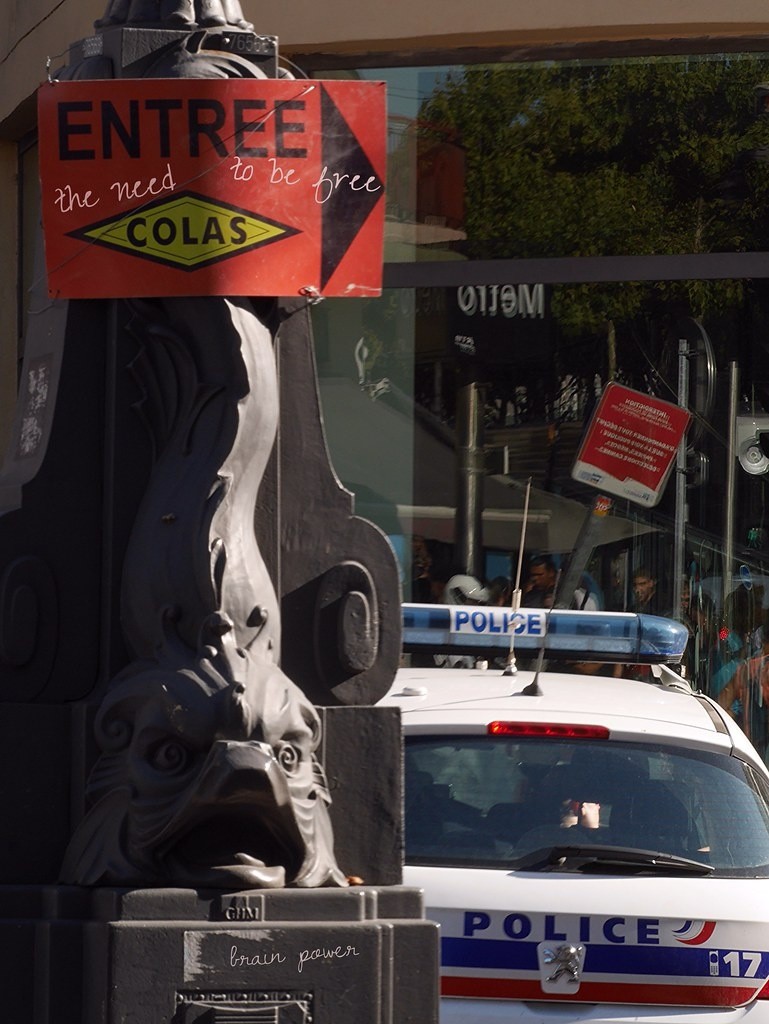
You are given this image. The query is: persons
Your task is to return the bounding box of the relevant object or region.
[401,535,769,769]
[403,754,706,865]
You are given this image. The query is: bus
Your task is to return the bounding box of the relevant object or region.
[524,529,769,768]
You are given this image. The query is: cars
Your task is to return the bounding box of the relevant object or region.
[396,600,769,1023]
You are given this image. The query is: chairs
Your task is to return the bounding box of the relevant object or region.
[404,746,712,868]
